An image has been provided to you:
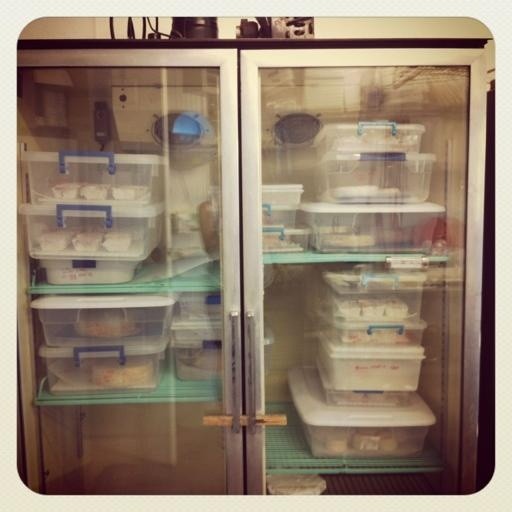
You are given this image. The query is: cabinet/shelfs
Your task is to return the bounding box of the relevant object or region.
[17,39,490,495]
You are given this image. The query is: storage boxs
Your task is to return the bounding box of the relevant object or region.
[315,342,427,394]
[311,120,426,156]
[175,289,224,322]
[17,201,166,263]
[169,319,224,383]
[36,341,171,395]
[315,149,437,205]
[261,223,311,253]
[37,258,138,285]
[285,363,437,459]
[31,293,179,353]
[260,203,299,229]
[20,146,167,210]
[315,320,428,354]
[297,200,448,253]
[260,182,305,211]
[317,268,428,326]
